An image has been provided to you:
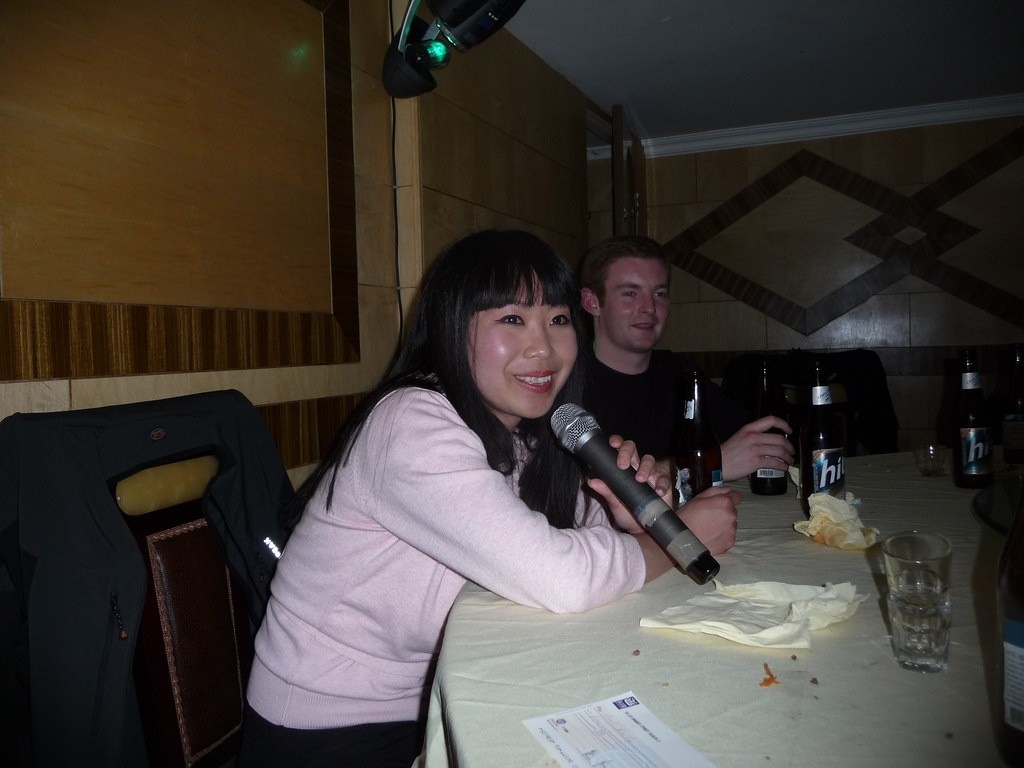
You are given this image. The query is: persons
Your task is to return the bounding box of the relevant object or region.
[244,224,746,768]
[573,235,799,510]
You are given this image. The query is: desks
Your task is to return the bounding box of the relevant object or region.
[412,444,1024,768]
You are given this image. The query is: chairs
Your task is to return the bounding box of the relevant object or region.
[0,343,1024,768]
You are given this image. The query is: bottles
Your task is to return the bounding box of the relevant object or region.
[674,371,722,507]
[798,360,845,516]
[994,475,1024,767]
[1001,349,1024,463]
[750,362,787,495]
[953,351,995,488]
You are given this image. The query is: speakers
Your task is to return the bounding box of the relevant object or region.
[427,0,526,53]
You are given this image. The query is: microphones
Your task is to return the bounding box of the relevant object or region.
[551,402,720,585]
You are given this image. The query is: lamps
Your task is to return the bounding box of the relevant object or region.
[382,0,527,98]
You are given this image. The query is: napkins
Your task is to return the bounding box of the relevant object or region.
[640,579,870,648]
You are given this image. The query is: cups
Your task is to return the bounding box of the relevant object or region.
[881,529,952,606]
[914,445,953,476]
